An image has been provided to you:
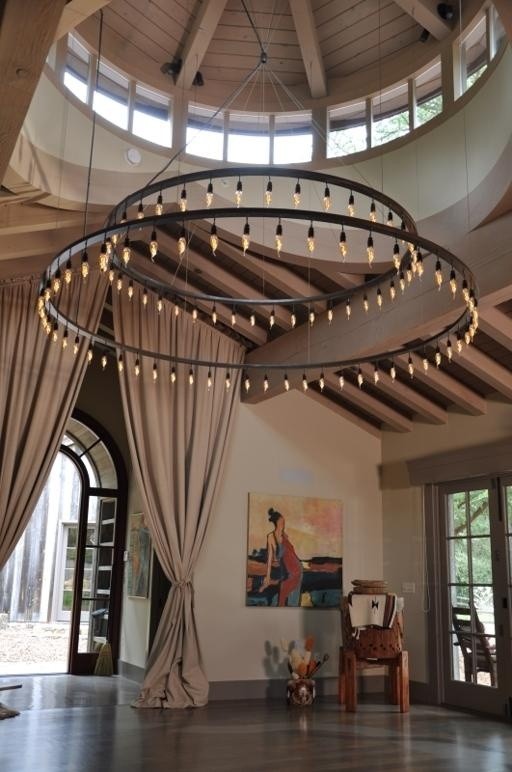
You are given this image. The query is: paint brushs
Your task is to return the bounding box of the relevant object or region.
[288,638,329,679]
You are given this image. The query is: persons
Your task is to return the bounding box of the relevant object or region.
[258,507,303,605]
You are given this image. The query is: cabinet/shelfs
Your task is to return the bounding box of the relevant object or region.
[87,496,118,652]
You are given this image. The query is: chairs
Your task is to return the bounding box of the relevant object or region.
[452,602,498,687]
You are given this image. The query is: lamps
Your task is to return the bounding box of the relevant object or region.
[36,1,480,394]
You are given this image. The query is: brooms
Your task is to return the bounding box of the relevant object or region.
[91,588,115,675]
